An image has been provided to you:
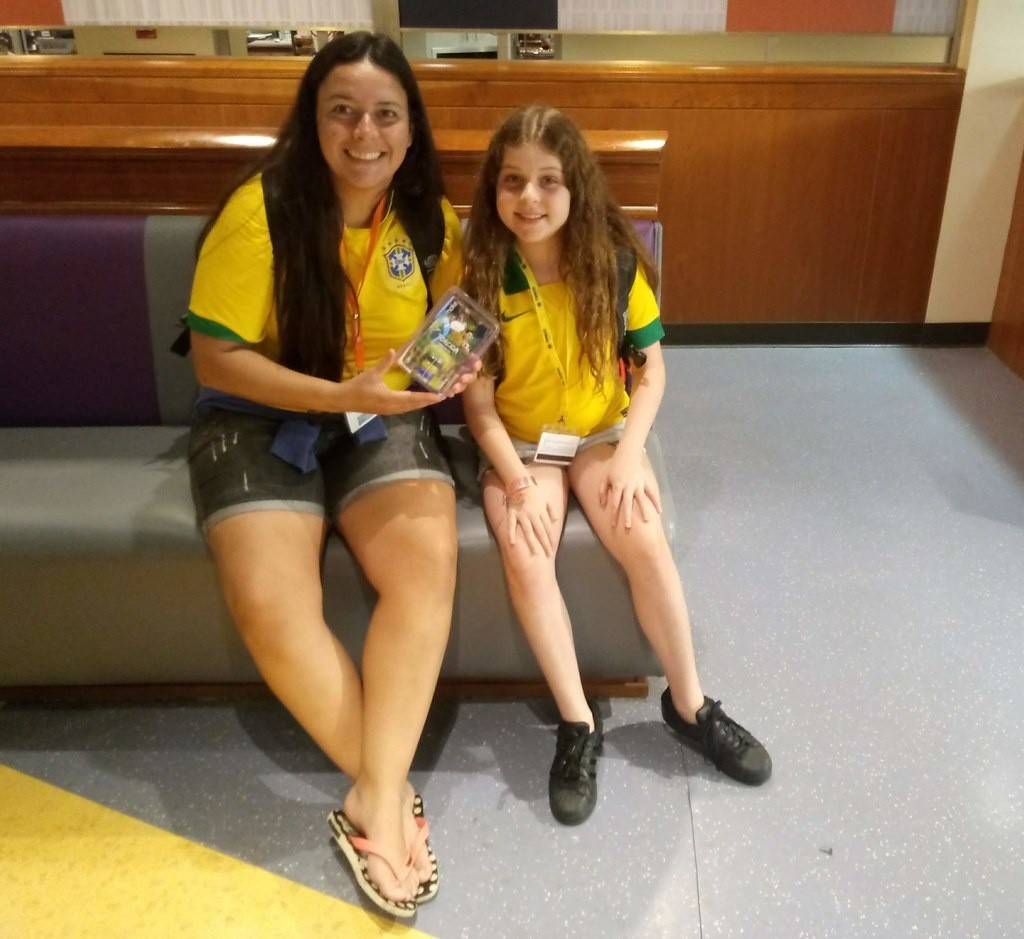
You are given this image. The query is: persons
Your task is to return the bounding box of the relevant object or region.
[451,104,774,827]
[184,30,483,918]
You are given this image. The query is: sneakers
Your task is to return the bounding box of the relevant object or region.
[548,699,603,825]
[661,683,772,787]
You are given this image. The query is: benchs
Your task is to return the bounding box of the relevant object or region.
[0,127,665,701]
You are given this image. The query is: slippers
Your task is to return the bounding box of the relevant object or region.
[407,794,441,903]
[326,810,417,918]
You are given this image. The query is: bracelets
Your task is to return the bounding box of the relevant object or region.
[505,474,538,496]
